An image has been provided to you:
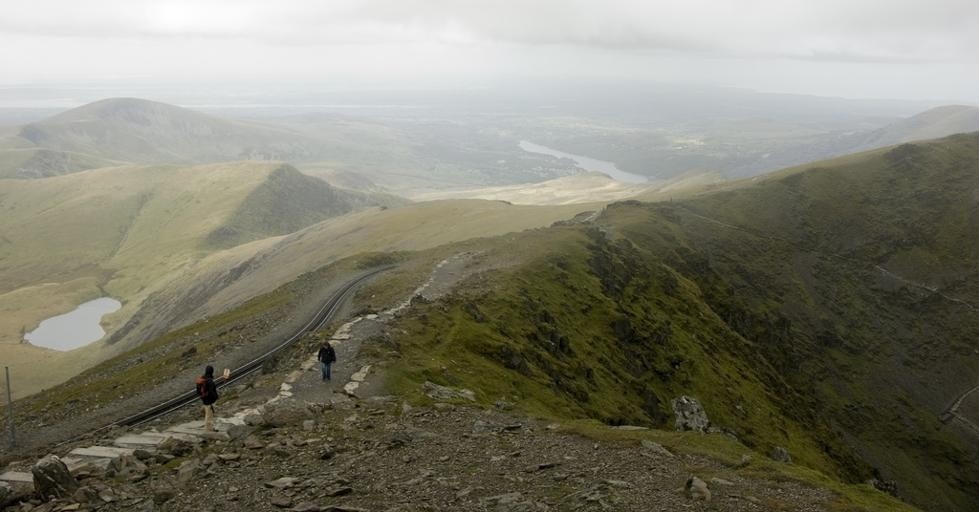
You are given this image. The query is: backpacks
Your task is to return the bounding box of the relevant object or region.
[196,377,212,397]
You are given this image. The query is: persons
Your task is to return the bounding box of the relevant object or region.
[196,364,219,432]
[317,341,336,381]
